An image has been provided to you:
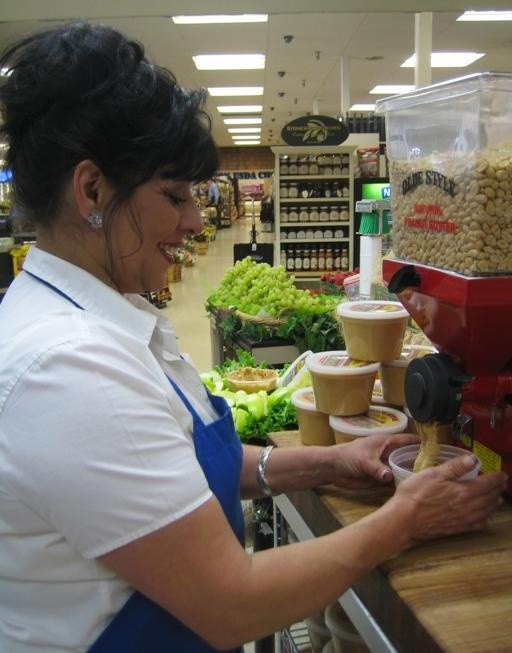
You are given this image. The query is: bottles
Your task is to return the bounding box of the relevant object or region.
[357,147,380,179]
[279,155,349,272]
[1,168,31,279]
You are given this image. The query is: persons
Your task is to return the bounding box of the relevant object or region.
[207,178,222,230]
[0,23,510,651]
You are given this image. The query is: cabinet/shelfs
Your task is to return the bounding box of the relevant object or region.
[261,430,512,652]
[274,145,354,282]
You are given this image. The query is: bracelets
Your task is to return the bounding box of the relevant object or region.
[255,444,274,501]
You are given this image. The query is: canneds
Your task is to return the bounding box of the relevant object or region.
[279,153,349,273]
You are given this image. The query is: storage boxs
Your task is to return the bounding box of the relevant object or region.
[373,70,512,279]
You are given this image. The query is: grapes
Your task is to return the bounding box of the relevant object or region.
[214,254,338,317]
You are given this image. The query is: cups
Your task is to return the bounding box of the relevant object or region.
[290,294,482,532]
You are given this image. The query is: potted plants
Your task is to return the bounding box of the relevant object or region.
[167,224,216,284]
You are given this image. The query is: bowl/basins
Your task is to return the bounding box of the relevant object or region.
[227,368,278,393]
